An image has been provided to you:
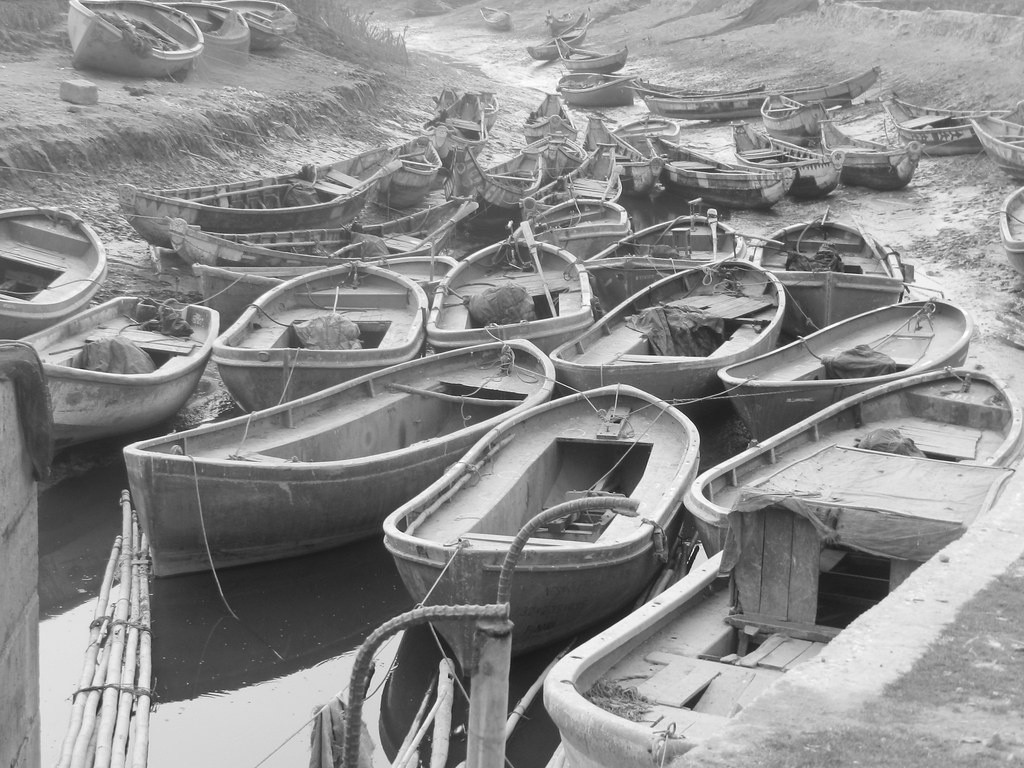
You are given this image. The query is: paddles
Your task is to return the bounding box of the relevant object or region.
[708,208,719,262]
[407,200,479,251]
[331,160,403,201]
[601,165,624,201]
[520,220,558,318]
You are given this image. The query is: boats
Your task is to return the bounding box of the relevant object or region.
[552,34,630,72]
[224,2,314,49]
[473,4,514,33]
[155,2,252,60]
[0,71,1023,768]
[57,0,205,71]
[521,13,593,61]
